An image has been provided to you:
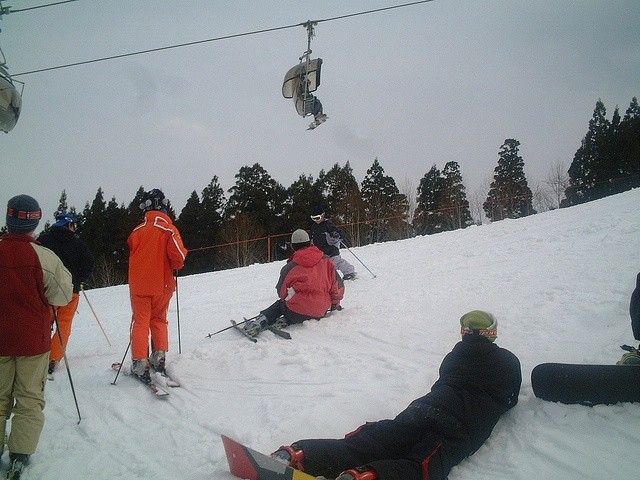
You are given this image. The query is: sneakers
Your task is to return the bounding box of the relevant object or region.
[616,344,640,364]
[243,314,271,337]
[335,465,377,480]
[343,272,357,280]
[150,350,165,372]
[6,453,30,480]
[271,316,287,330]
[48,360,55,374]
[270,442,304,472]
[315,114,327,121]
[130,359,151,383]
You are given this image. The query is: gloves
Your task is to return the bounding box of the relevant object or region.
[331,304,336,311]
[332,231,340,238]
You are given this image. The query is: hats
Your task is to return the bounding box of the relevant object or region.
[460,309,497,343]
[6,194,42,233]
[52,214,75,226]
[311,206,323,216]
[151,203,168,215]
[291,229,310,244]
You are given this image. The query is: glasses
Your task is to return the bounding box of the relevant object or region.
[310,213,325,221]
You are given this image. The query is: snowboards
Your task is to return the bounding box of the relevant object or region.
[530,363,640,405]
[221,435,321,480]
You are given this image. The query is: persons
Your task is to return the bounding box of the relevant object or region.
[300,78,328,123]
[268,309,522,479]
[126,188,188,383]
[0,194,76,479]
[34,213,90,379]
[285,206,359,281]
[240,229,345,338]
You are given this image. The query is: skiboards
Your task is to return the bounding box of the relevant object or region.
[111,361,180,396]
[230,317,292,344]
